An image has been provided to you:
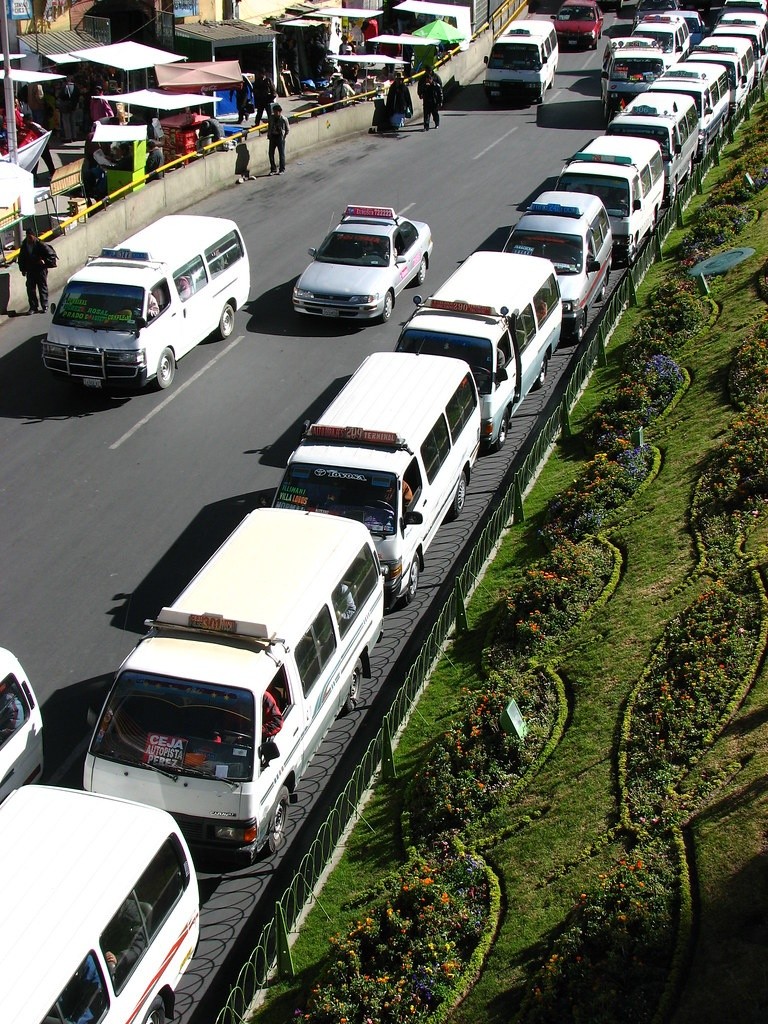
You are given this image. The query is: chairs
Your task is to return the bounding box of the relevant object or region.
[515,287,550,349]
[130,899,152,937]
[301,581,358,666]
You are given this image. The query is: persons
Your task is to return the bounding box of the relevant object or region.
[229,75,253,124]
[474,346,506,371]
[525,291,546,322]
[267,105,290,176]
[175,275,190,301]
[62,951,118,1024]
[210,248,225,275]
[331,35,361,110]
[0,82,134,210]
[417,65,443,132]
[251,69,276,128]
[385,72,413,131]
[379,477,413,507]
[333,584,357,619]
[145,141,164,184]
[134,290,160,321]
[361,16,401,79]
[199,119,224,143]
[222,689,283,735]
[586,249,595,267]
[19,228,53,315]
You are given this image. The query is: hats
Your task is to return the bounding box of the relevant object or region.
[334,76,348,86]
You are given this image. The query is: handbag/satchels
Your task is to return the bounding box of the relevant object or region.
[247,98,254,113]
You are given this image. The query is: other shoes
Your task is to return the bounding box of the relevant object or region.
[420,128,428,132]
[269,172,276,175]
[435,125,439,129]
[278,172,284,175]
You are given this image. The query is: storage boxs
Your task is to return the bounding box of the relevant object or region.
[51,213,78,235]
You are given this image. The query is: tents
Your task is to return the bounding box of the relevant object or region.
[153,60,245,115]
[175,18,283,92]
[0,69,67,98]
[68,41,189,123]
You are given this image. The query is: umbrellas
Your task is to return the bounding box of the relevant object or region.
[327,54,410,102]
[412,19,467,44]
[364,34,442,76]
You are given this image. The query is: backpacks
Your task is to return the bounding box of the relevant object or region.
[37,239,59,268]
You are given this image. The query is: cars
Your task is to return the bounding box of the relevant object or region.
[550,0,604,49]
[289,203,437,327]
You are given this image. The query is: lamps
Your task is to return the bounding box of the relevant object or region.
[500,698,525,739]
[743,172,754,187]
[630,427,644,447]
[697,272,710,296]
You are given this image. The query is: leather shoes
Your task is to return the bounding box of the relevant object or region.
[43,306,49,313]
[26,307,38,315]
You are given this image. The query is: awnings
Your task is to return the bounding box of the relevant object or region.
[279,19,332,27]
[47,54,90,64]
[91,88,224,120]
[302,8,384,18]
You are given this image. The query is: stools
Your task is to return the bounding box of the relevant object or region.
[68,197,86,215]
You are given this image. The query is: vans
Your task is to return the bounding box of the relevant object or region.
[270,351,483,606]
[0,782,202,1024]
[395,0,768,445]
[0,647,45,806]
[81,508,384,863]
[42,214,251,390]
